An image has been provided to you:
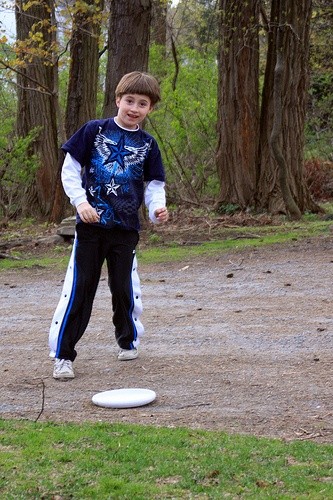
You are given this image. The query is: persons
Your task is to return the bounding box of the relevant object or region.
[48,71,168,379]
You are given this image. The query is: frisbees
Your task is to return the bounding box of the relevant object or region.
[91,388,156,408]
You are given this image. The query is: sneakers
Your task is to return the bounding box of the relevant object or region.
[118,348,138,360]
[51,358,74,378]
